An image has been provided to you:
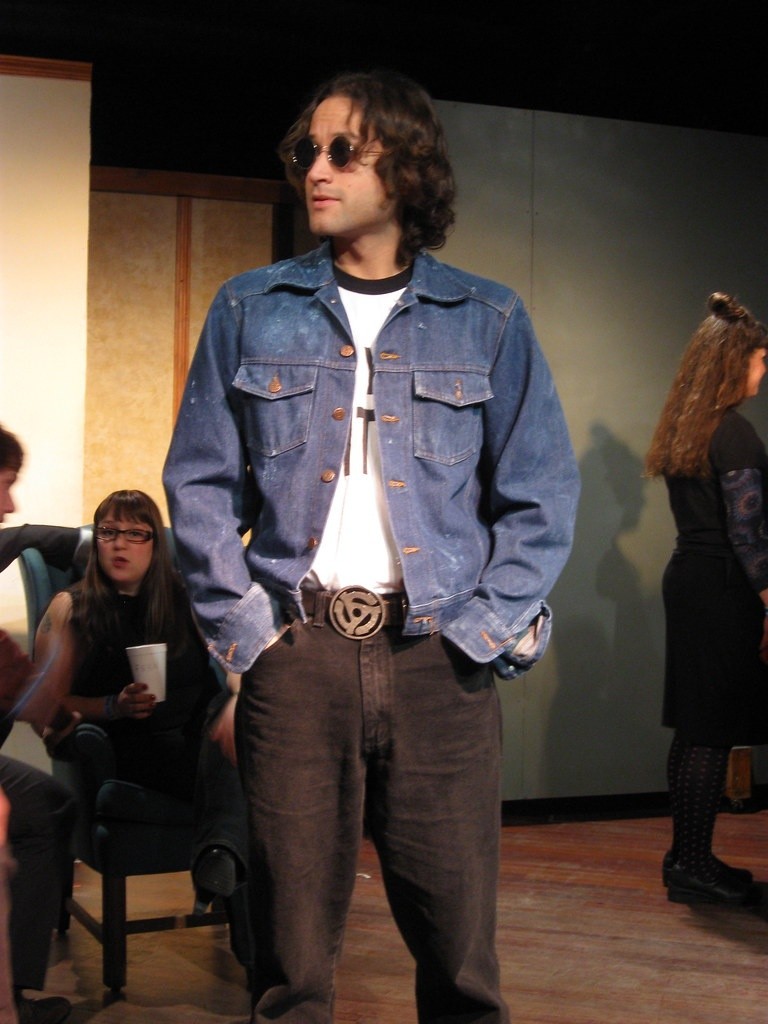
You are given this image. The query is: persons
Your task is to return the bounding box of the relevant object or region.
[33,488,297,1024]
[0,426,83,1024]
[158,63,586,1024]
[639,288,768,909]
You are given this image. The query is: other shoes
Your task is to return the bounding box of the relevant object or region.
[16,994,72,1024]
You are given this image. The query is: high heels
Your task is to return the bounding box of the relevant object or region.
[668,866,762,904]
[662,853,753,886]
[194,846,236,916]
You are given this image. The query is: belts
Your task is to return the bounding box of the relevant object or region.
[300,588,409,639]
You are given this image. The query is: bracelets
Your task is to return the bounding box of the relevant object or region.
[103,694,119,718]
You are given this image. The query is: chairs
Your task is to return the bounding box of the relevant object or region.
[21,541,252,992]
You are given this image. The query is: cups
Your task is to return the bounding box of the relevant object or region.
[125,643,167,702]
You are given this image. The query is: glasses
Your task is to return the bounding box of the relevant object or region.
[291,137,387,168]
[92,527,154,543]
